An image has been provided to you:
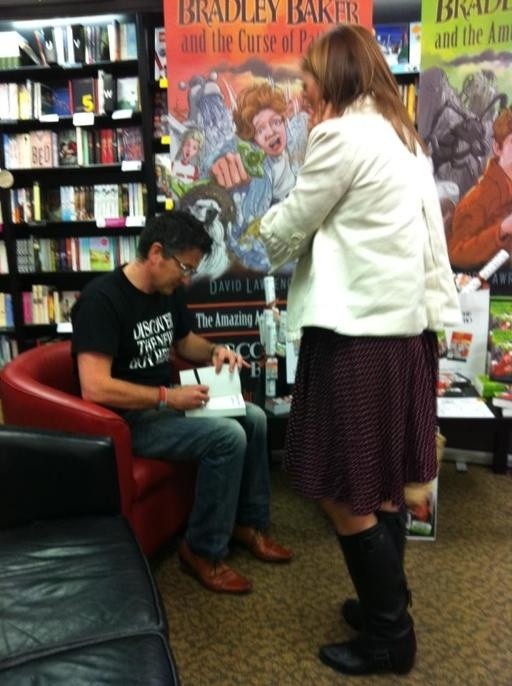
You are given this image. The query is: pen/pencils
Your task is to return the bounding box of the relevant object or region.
[191,362,202,387]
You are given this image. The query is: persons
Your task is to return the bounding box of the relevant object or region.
[447,105,512,266]
[210,78,312,273]
[170,128,205,188]
[70,208,295,594]
[241,24,462,676]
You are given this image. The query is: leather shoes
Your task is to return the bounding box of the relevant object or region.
[178,538,253,593]
[232,523,294,564]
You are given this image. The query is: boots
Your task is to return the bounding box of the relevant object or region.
[319,522,416,675]
[341,509,408,634]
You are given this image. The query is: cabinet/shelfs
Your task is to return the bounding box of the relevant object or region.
[0,0,150,360]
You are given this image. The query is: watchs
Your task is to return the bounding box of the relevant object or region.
[157,384,169,413]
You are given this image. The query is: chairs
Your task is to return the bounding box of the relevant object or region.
[0,429,181,686]
[0,337,190,573]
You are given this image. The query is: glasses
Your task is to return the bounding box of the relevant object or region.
[173,253,199,279]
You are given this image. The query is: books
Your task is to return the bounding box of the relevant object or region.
[0,336,54,367]
[2,74,139,120]
[5,178,149,228]
[178,362,249,418]
[0,17,143,70]
[1,124,141,166]
[2,283,78,326]
[1,234,138,269]
